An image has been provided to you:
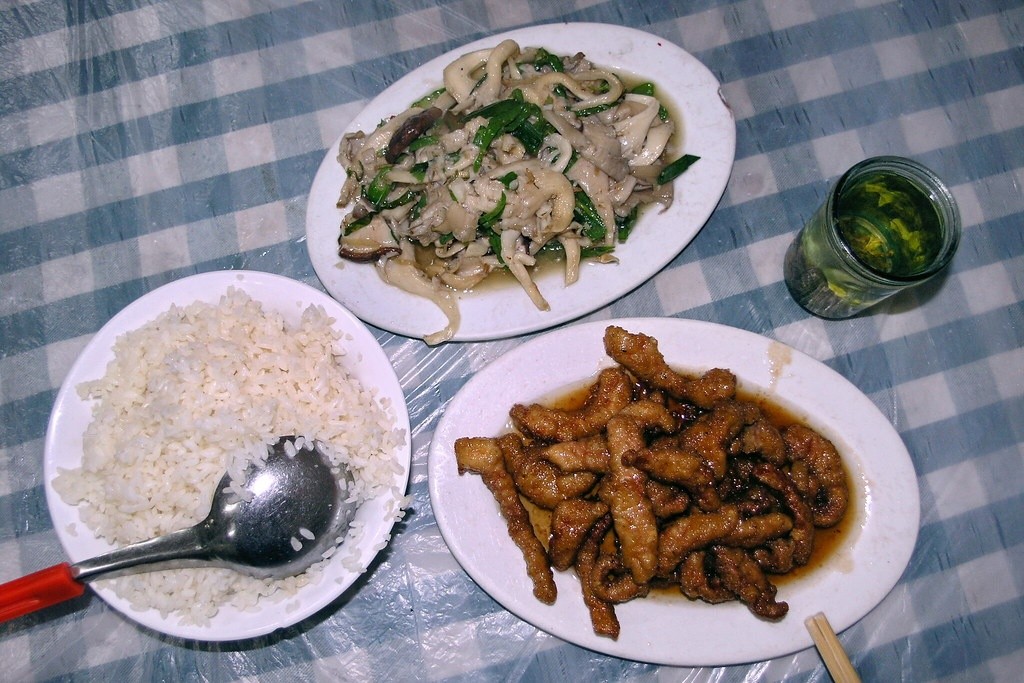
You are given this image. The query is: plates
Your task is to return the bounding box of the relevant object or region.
[427,315,923,668]
[42,269,413,641]
[304,19,738,342]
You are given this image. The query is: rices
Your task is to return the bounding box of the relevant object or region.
[52,274,420,629]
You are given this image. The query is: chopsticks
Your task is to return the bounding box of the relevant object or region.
[803,609,865,683]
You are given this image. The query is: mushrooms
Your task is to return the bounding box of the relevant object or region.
[335,40,676,349]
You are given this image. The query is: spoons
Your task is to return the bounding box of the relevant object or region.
[0,435,360,630]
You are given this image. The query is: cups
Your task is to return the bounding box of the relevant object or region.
[781,152,961,323]
